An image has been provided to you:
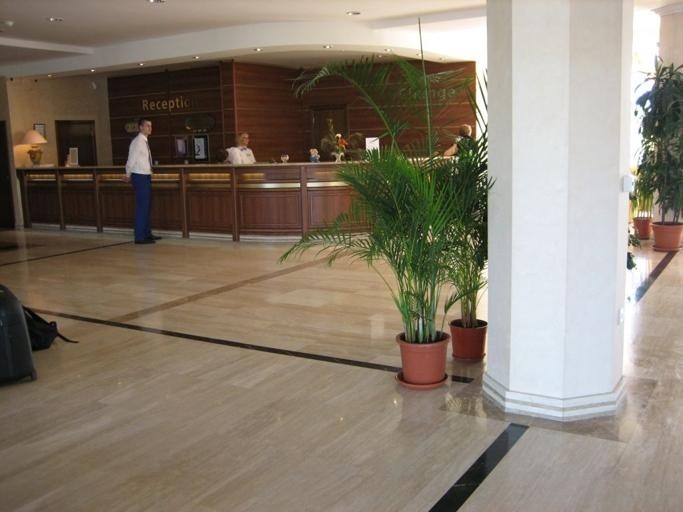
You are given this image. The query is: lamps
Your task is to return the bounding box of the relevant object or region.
[17,128,47,167]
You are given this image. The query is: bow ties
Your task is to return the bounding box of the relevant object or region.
[241,146,246,151]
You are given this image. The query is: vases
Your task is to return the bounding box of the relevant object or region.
[311,154,319,163]
[331,149,343,163]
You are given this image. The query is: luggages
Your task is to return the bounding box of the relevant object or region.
[0,285,38,386]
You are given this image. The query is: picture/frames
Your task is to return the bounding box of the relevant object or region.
[31,122,45,139]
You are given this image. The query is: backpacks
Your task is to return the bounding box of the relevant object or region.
[23,304,79,350]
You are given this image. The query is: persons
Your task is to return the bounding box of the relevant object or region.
[442,124,481,213]
[211,148,232,165]
[238,129,257,163]
[224,131,252,165]
[122,118,163,245]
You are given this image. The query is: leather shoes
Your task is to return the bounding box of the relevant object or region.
[135,239,156,244]
[151,235,161,240]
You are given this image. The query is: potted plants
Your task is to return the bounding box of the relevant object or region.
[630,152,652,241]
[630,56,683,253]
[279,129,451,389]
[287,16,488,362]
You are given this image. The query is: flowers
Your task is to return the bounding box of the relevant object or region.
[308,148,318,156]
[317,124,361,153]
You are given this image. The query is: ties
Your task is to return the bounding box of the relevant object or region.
[146,141,156,170]
